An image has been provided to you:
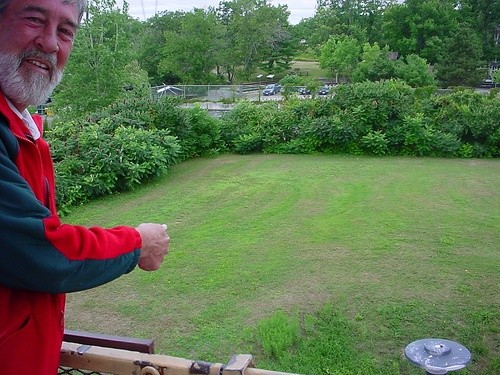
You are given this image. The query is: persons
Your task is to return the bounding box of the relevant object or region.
[0,0,170,375]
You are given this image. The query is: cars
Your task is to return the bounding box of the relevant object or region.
[318,87,330,95]
[299,87,311,95]
[280,87,298,95]
[263,84,280,96]
